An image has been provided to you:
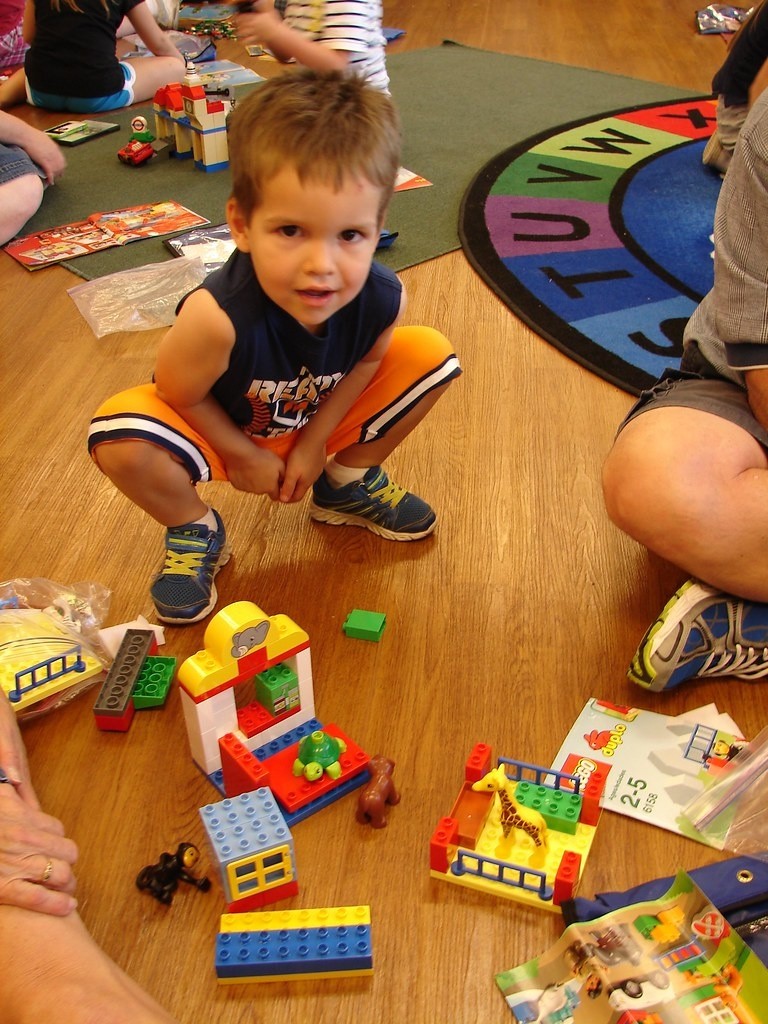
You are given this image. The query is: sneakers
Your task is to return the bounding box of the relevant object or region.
[310,466,437,540]
[150,508,233,624]
[627,576,768,693]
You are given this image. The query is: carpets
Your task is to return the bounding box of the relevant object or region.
[456,93,725,398]
[14,36,710,332]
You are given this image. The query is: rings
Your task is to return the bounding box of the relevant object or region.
[42,862,52,882]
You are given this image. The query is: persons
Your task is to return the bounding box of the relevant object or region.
[0,110,66,247]
[238,0,390,97]
[88,69,461,624]
[0,685,178,1024]
[0,0,30,110]
[602,0,768,694]
[23,0,185,114]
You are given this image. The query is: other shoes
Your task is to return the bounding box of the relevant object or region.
[702,128,732,172]
